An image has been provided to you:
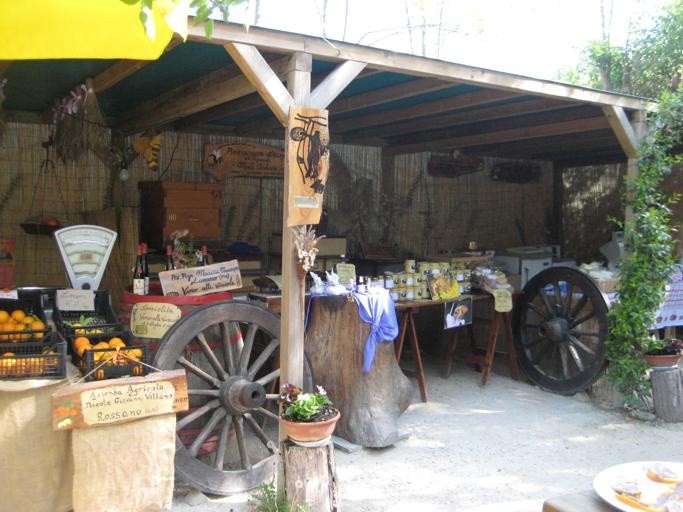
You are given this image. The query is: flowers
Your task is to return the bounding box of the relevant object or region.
[278,383,335,419]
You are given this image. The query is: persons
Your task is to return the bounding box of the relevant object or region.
[446,301,469,329]
[305,131,321,178]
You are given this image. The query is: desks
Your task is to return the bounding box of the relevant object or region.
[249,288,527,433]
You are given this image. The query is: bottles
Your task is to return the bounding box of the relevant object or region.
[139,243,149,296]
[132,246,144,296]
[199,246,208,265]
[339,253,345,264]
[356,275,391,296]
[163,244,174,271]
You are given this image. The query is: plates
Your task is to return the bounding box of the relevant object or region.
[594,460,683,512]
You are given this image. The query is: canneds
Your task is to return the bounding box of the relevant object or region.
[374,259,471,301]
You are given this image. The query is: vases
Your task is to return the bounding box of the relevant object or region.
[279,408,341,441]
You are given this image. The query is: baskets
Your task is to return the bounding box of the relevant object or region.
[0,290,147,380]
